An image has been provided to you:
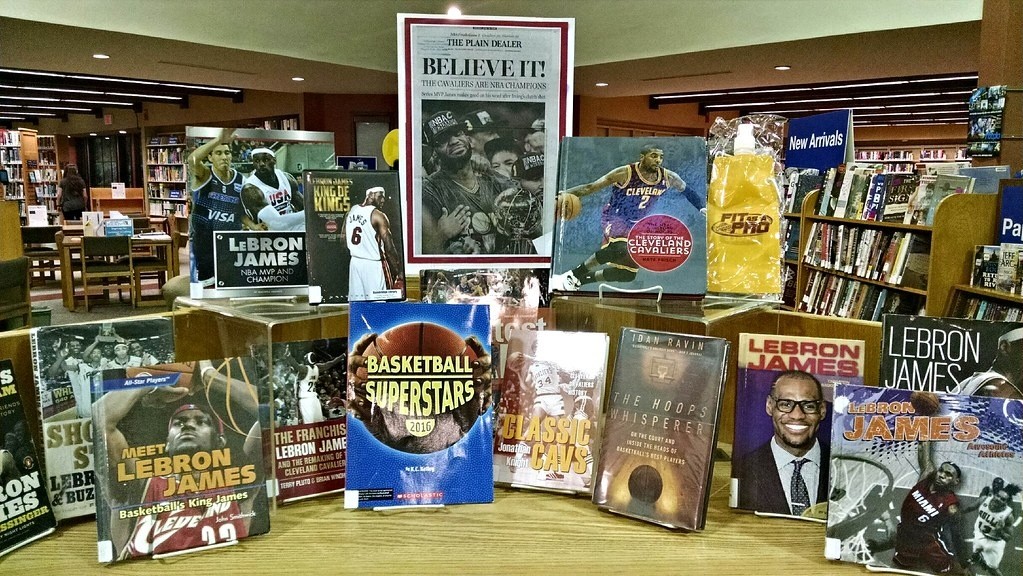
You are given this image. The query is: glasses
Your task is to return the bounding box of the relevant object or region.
[775,398,821,416]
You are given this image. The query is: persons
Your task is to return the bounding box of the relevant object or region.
[887,418,972,576]
[978,477,1005,498]
[55,164,87,220]
[951,327,1023,400]
[0,110,830,562]
[960,484,1023,576]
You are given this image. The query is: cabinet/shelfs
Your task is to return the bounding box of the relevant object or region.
[781,168,1023,323]
[87,186,148,224]
[145,113,304,239]
[0,128,64,221]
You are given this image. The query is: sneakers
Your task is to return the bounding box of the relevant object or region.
[551,270,581,299]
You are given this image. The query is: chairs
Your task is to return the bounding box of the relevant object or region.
[61,232,174,309]
[114,229,183,298]
[0,256,35,333]
[19,225,65,289]
[80,235,137,311]
[54,229,111,301]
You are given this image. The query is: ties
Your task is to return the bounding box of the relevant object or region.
[789,458,810,515]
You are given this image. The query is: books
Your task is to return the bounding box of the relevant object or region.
[28,316,177,526]
[143,310,1023,576]
[0,127,1023,323]
[0,358,58,558]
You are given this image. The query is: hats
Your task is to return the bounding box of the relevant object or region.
[114,338,128,352]
[516,150,544,178]
[460,107,510,136]
[483,137,525,161]
[424,109,463,144]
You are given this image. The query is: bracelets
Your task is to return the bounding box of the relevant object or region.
[56,205,60,207]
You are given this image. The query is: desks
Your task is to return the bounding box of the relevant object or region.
[0,302,1023,576]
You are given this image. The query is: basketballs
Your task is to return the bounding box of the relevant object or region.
[909,391,940,416]
[354,320,485,455]
[125,359,197,406]
[627,464,664,503]
[507,352,526,372]
[557,192,581,221]
